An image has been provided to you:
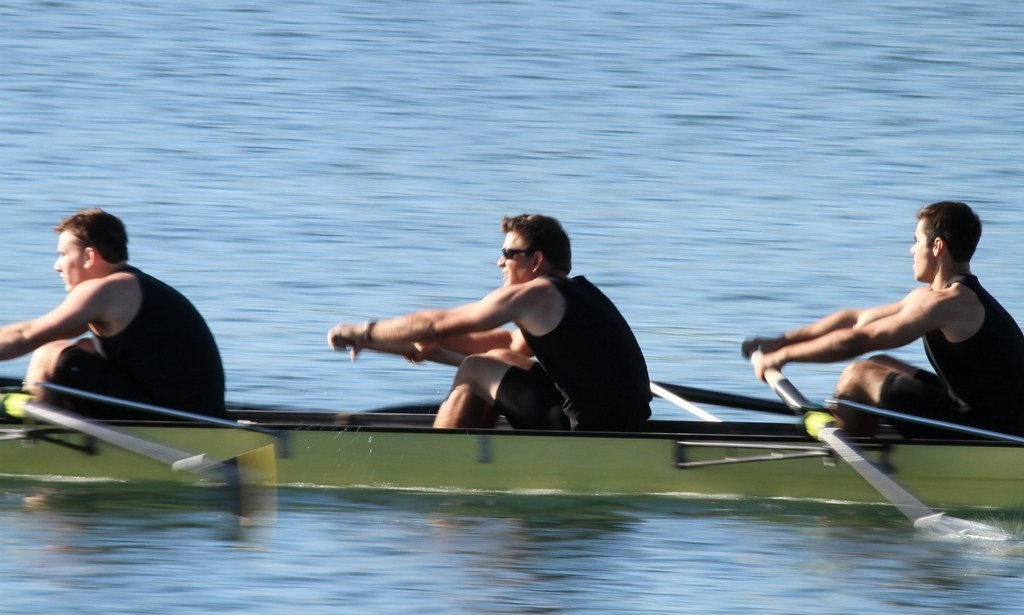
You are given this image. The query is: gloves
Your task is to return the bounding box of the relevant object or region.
[742,334,788,360]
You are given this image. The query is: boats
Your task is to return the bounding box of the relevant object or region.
[0,377,1023,522]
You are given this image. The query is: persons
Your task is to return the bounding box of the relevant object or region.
[741,201,1024,450]
[327,213,653,432]
[0,207,227,426]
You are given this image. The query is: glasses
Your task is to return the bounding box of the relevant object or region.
[501,248,530,259]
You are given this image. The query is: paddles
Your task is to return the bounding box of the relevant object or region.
[331,330,833,422]
[744,346,1013,545]
[0,391,262,488]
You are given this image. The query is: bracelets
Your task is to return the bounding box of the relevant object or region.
[364,320,376,341]
